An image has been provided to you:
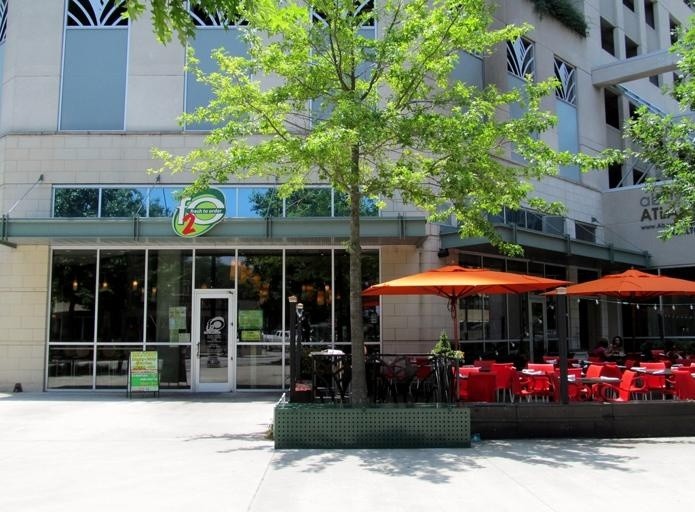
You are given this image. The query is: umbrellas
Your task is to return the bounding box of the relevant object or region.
[538,265,695,401]
[360,257,575,402]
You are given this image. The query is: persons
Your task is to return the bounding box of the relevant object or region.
[294,303,311,349]
[609,336,625,355]
[589,336,612,362]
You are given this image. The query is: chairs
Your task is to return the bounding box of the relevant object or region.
[335,346,695,403]
